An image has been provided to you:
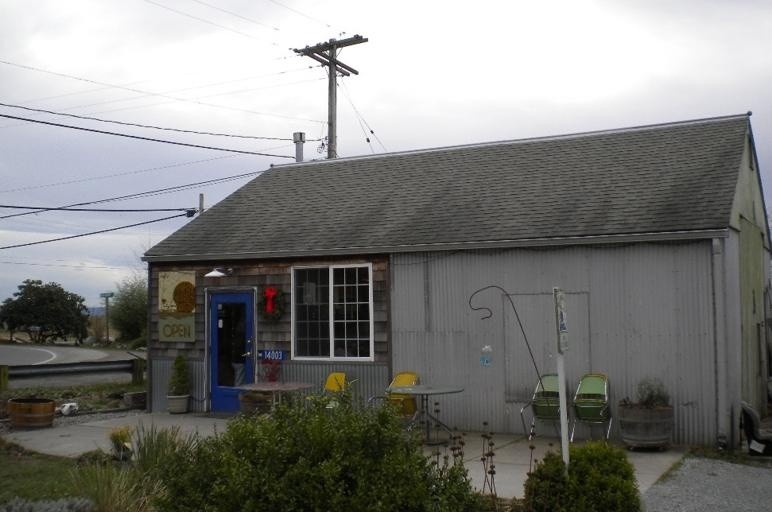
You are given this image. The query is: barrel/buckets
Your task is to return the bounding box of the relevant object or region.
[7,398,54,428]
[618,403,674,449]
[240,402,270,414]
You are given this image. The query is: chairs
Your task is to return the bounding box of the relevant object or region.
[305,371,419,434]
[519,372,613,444]
[742,400,772,457]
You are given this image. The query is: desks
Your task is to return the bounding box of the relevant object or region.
[386,386,464,445]
[239,382,314,412]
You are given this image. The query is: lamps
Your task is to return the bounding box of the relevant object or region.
[204,267,234,278]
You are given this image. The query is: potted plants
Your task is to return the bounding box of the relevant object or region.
[107,422,137,462]
[166,351,193,413]
[617,373,674,452]
